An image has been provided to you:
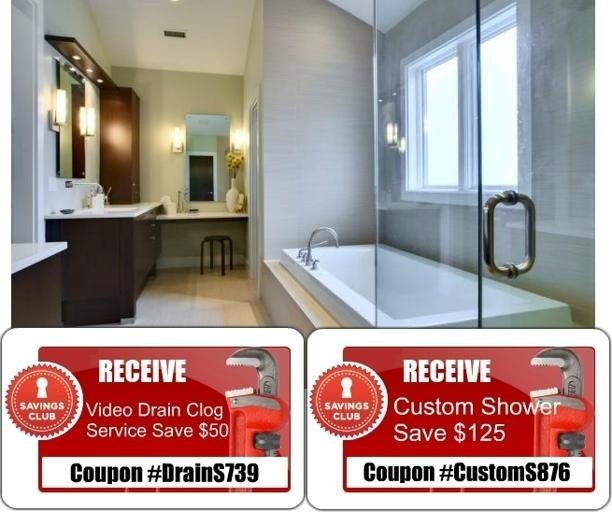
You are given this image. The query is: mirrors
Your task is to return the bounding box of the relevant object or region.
[184,111,229,204]
[56,59,85,179]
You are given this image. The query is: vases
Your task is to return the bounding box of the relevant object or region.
[225,179,244,214]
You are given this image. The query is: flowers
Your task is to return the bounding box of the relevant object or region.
[226,147,243,180]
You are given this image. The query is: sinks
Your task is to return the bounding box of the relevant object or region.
[73,207,139,213]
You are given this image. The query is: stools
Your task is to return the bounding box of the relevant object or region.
[201,236,233,278]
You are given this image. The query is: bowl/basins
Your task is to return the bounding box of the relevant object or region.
[60,210,74,214]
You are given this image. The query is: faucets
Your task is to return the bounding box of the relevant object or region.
[74,182,104,194]
[307,226,341,263]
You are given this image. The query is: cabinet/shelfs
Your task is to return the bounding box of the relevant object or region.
[46,207,162,323]
[101,88,139,205]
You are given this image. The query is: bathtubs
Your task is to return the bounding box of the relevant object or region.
[280,242,584,329]
